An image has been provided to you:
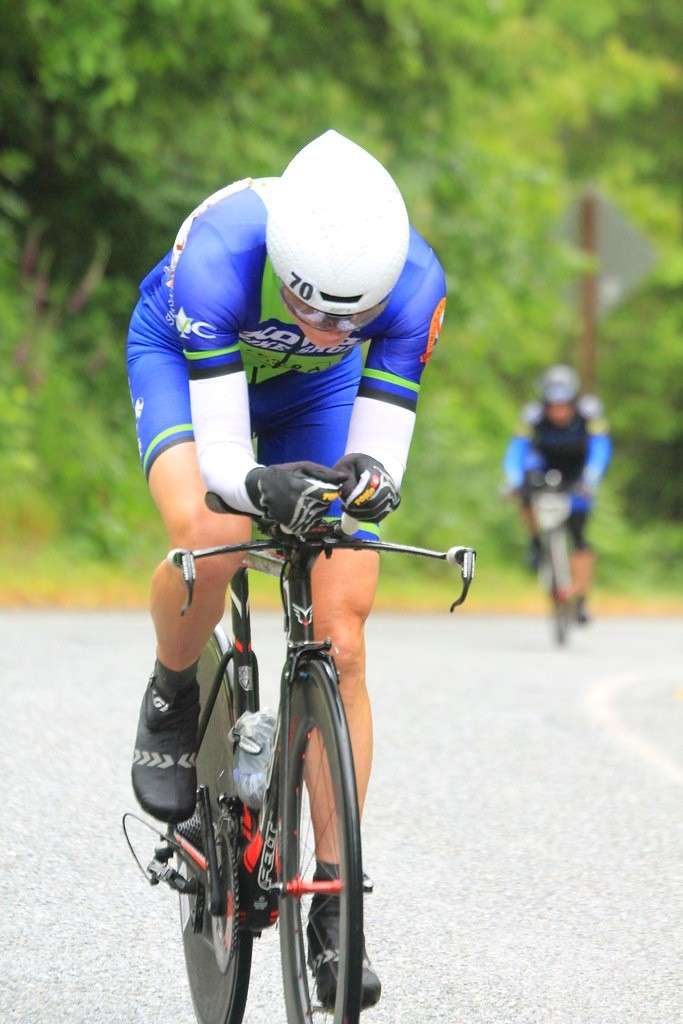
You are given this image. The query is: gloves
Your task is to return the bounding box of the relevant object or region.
[245,461,350,534]
[332,452,402,524]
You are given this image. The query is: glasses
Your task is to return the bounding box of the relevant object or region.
[279,286,383,334]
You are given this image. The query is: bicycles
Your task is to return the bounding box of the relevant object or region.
[510,463,586,648]
[120,490,477,1024]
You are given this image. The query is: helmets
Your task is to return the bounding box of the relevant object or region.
[265,128,412,315]
[542,365,579,404]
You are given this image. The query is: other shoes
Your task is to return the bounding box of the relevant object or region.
[575,595,588,623]
[130,671,202,825]
[523,538,543,575]
[306,876,381,1010]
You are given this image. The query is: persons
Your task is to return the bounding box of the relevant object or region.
[503,362,613,624]
[124,129,447,1015]
